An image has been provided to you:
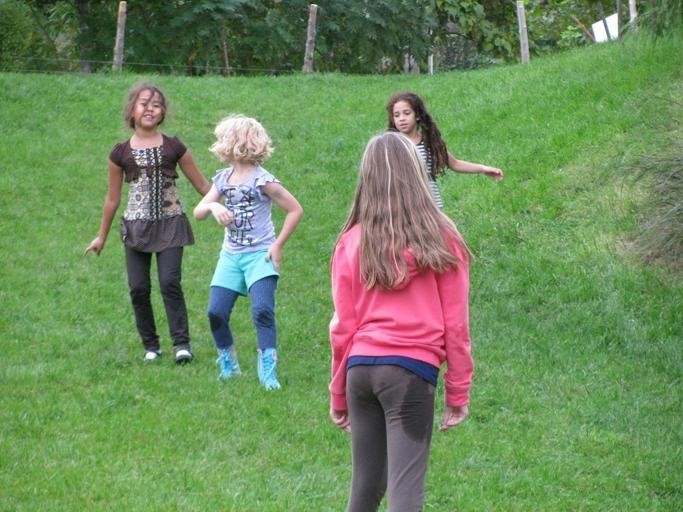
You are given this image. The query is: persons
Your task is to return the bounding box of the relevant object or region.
[83,82,212,364]
[386,90,503,209]
[325,130,475,512]
[194,113,303,392]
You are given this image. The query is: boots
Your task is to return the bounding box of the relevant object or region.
[257,349,282,391]
[216,346,240,381]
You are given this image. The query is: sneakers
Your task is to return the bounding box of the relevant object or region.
[144,350,162,360]
[174,349,193,364]
